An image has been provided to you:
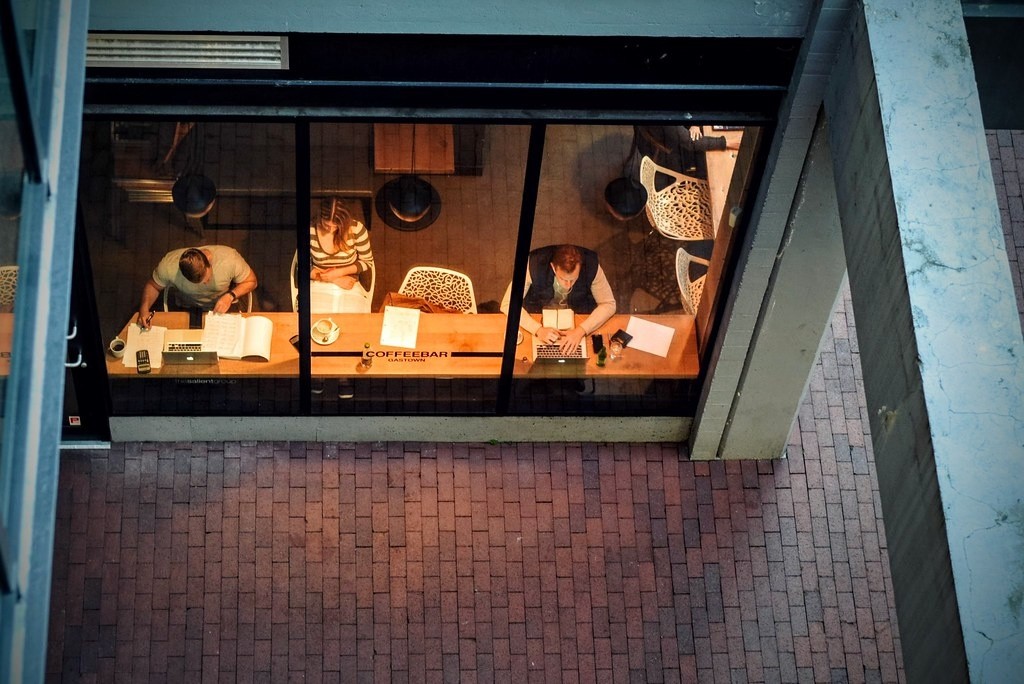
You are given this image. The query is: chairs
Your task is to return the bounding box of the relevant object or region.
[675,248,711,316]
[163,278,253,313]
[0,265,18,312]
[639,155,715,241]
[290,248,376,314]
[398,266,478,315]
[625,125,676,188]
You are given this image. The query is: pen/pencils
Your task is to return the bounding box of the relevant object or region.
[141,310,155,330]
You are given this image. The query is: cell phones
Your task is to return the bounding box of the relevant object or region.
[592,335,603,355]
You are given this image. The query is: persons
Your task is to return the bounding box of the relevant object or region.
[138,245,257,329]
[633,124,741,174]
[310,195,374,290]
[500,244,616,355]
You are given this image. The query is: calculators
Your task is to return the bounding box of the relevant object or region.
[136,350,152,375]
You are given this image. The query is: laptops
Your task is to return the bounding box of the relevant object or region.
[162,342,219,365]
[531,335,590,365]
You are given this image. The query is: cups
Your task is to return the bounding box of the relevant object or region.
[110,336,126,358]
[611,342,622,360]
[317,320,332,334]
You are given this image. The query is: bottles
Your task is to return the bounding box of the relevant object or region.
[361,343,372,368]
[596,346,607,366]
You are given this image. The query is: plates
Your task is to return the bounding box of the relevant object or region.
[504,330,523,345]
[310,322,338,344]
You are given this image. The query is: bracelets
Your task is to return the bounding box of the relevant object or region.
[227,290,236,301]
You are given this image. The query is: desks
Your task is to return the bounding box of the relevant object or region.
[194,121,377,232]
[702,124,746,244]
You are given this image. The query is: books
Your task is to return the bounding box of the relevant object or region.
[204,315,273,361]
[542,309,575,331]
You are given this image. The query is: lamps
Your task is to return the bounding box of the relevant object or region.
[171,122,216,219]
[605,121,648,221]
[387,121,433,222]
